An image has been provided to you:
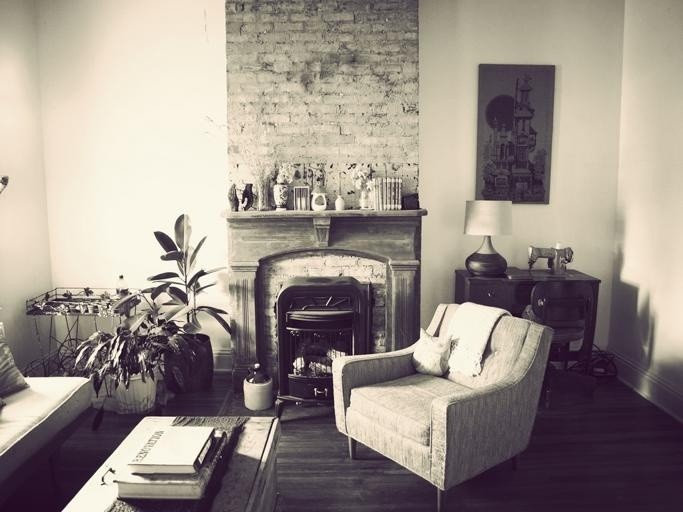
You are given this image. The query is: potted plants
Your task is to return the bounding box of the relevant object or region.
[71,208,239,432]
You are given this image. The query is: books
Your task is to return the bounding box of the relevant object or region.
[111,429,227,500]
[126,426,216,474]
[371,177,403,210]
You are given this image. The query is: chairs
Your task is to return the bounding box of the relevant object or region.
[332,300,555,512]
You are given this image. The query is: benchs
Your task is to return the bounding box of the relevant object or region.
[0,323,96,508]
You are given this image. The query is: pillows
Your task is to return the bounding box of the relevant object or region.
[409,327,454,376]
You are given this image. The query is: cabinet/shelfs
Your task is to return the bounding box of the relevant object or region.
[453,266,602,362]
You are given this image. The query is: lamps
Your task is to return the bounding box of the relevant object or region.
[461,198,515,278]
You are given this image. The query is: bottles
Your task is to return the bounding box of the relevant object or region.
[247,363,270,383]
[273,182,287,206]
[335,195,345,210]
